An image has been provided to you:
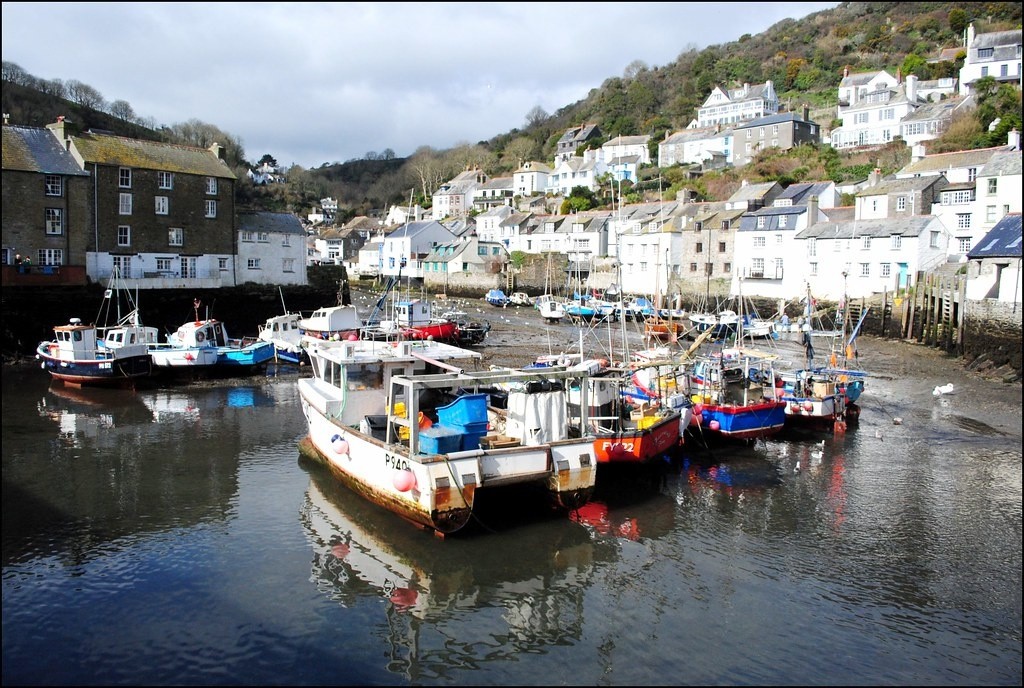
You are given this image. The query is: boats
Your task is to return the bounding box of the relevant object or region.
[485,227,843,341]
[35,264,487,390]
[298,330,597,540]
[487,134,692,470]
[705,276,870,434]
[623,171,787,446]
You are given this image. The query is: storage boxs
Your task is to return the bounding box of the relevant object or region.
[815,383,834,396]
[630,392,684,430]
[385,393,520,454]
[659,377,676,388]
[840,375,847,382]
[692,395,711,404]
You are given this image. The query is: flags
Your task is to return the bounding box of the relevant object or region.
[844,345,853,360]
[831,354,835,367]
[812,298,816,305]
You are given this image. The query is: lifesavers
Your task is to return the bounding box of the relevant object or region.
[197,332,205,341]
[215,325,221,334]
[834,421,847,434]
[593,288,604,296]
[601,365,606,369]
[712,351,721,358]
[178,330,185,341]
[593,357,608,365]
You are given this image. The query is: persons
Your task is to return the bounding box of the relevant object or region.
[797,315,804,333]
[14,254,31,264]
[780,312,789,334]
